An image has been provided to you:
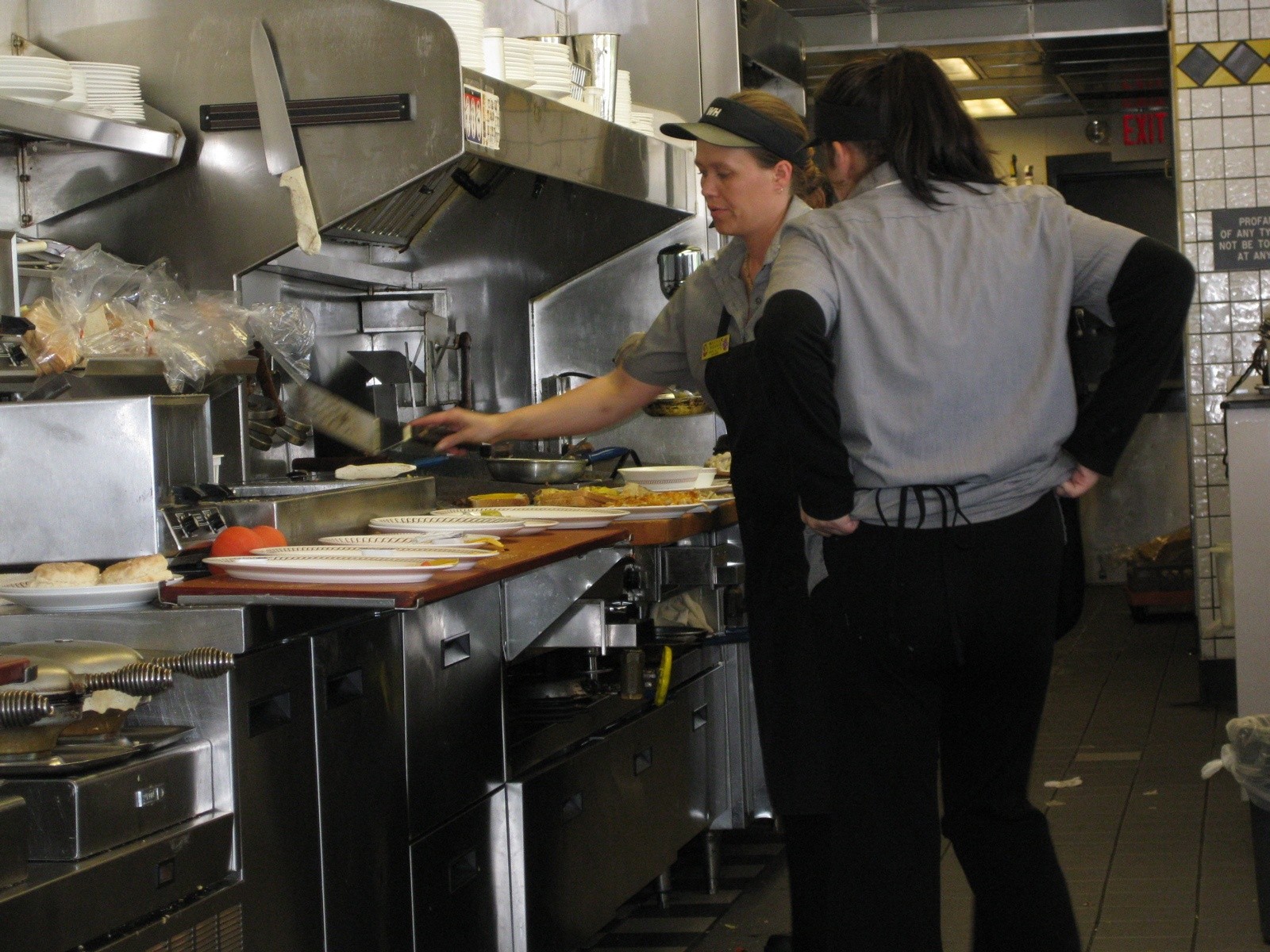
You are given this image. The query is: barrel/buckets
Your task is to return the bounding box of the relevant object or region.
[520,32,621,122]
[483,28,505,81]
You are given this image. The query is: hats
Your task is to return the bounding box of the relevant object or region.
[659,97,808,170]
[790,106,883,154]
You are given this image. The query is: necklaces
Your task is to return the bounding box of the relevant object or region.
[742,257,762,290]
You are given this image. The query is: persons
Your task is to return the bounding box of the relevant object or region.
[753,43,1195,952]
[410,91,828,952]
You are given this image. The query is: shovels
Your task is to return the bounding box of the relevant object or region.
[294,385,491,463]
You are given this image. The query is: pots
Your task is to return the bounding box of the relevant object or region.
[484,446,627,483]
[641,392,715,418]
[606,625,748,664]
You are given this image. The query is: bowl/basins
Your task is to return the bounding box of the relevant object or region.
[616,465,717,490]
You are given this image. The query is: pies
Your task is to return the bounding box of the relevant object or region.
[30,554,174,588]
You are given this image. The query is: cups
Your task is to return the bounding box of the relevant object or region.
[582,86,605,116]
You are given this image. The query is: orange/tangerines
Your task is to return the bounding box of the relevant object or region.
[205,525,286,574]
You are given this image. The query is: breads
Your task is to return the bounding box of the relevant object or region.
[16,304,83,375]
[468,488,619,509]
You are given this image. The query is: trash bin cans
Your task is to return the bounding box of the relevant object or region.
[1229,713,1269,945]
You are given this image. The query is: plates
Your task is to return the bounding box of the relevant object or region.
[391,0,486,73]
[602,503,702,521]
[430,506,630,530]
[250,545,498,570]
[368,515,527,536]
[686,498,735,512]
[615,69,654,136]
[514,519,558,535]
[0,55,146,125]
[201,556,454,583]
[0,574,184,609]
[503,37,572,102]
[318,534,500,548]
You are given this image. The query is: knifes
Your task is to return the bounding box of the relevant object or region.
[250,18,322,256]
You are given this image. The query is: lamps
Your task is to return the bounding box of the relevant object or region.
[1085,108,1174,163]
